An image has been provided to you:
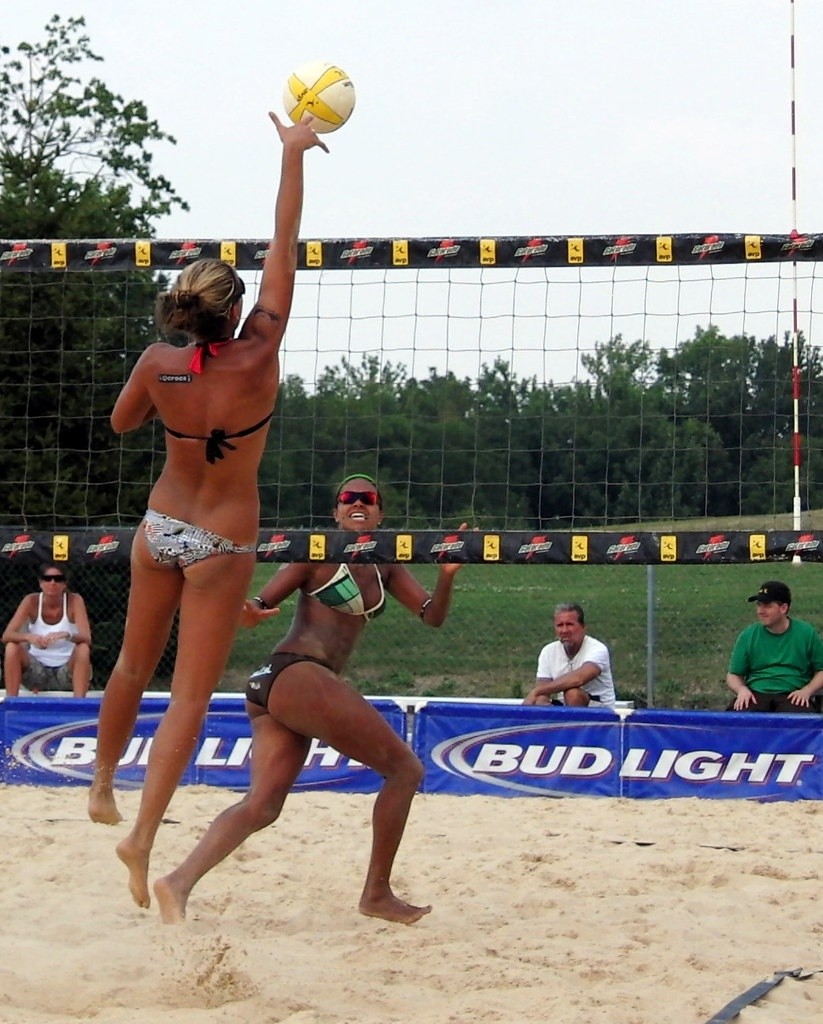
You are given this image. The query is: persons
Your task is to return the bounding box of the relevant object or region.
[2,559,91,699]
[725,581,823,712]
[86,112,330,910]
[521,601,616,714]
[155,474,481,925]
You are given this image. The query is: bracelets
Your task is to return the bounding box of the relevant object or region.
[420,598,432,618]
[66,632,73,641]
[253,596,268,610]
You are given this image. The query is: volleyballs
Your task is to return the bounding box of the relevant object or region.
[281,65,357,136]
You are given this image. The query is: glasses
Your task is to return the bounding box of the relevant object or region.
[41,575,65,582]
[231,277,245,309]
[335,491,382,510]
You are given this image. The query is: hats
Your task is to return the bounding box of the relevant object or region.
[747,581,791,606]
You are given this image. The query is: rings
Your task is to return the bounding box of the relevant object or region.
[312,128,315,132]
